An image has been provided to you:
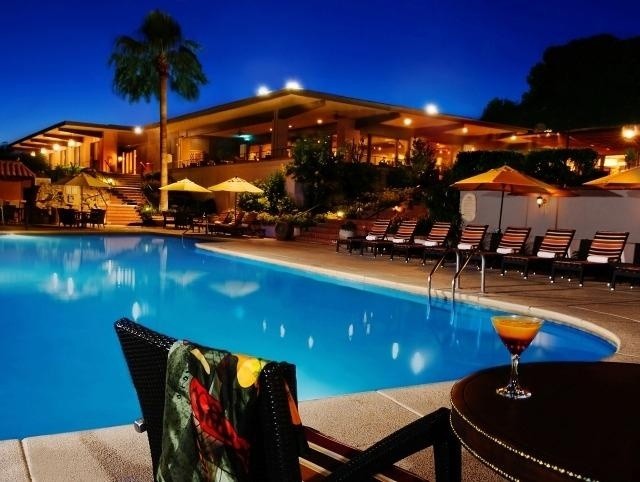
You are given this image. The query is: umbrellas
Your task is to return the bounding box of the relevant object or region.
[447,159,560,232]
[48,171,113,212]
[207,175,265,223]
[581,165,640,192]
[156,177,213,214]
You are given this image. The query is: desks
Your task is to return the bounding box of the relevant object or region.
[448,351,640,481]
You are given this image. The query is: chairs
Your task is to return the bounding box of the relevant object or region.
[463,227,532,273]
[161,208,261,237]
[500,227,577,281]
[392,222,453,266]
[112,317,465,482]
[423,223,489,270]
[339,218,389,260]
[361,219,419,260]
[551,230,630,290]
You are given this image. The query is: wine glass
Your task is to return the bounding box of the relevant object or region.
[490,315,545,398]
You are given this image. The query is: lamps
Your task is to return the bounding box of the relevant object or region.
[536,195,544,209]
[0,201,107,231]
